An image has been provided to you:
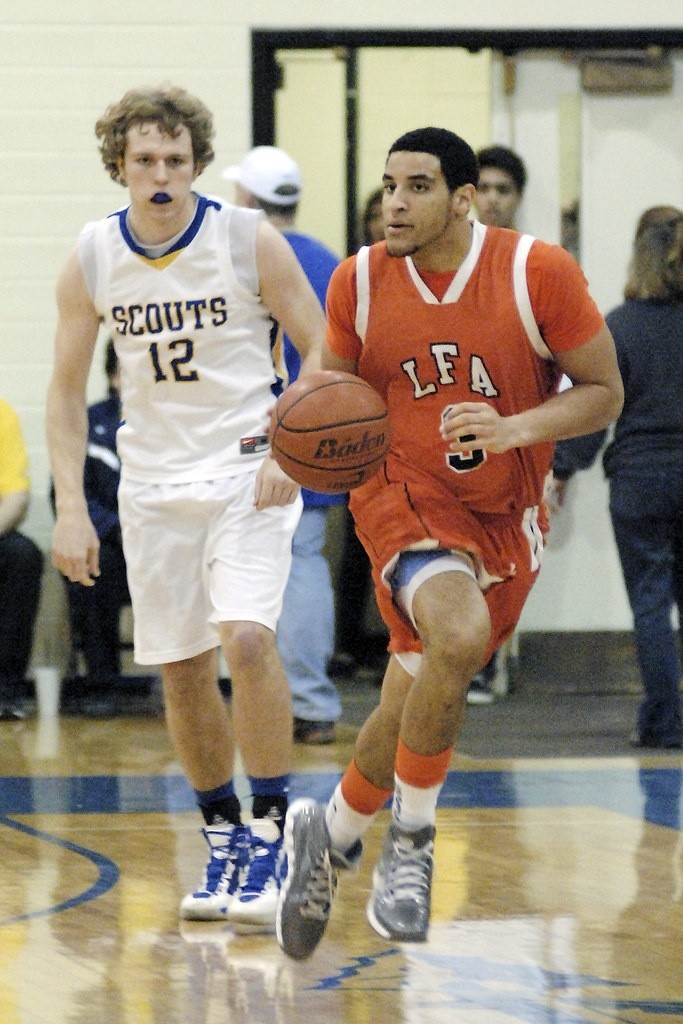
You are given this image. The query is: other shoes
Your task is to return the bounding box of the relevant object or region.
[466,654,499,708]
[293,714,340,747]
[629,691,682,754]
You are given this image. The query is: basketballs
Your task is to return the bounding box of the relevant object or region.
[270,370,388,494]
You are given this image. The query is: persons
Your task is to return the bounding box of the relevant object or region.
[537,205,683,747]
[45,86,331,925]
[0,400,44,719]
[221,145,342,746]
[466,144,525,704]
[49,338,133,718]
[264,128,624,961]
[327,189,390,685]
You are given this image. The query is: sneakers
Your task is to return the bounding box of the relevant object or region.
[226,813,290,938]
[366,821,438,944]
[181,821,235,923]
[275,795,366,964]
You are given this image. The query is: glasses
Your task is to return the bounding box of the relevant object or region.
[476,181,516,196]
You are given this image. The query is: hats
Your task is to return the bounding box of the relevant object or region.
[220,144,305,206]
